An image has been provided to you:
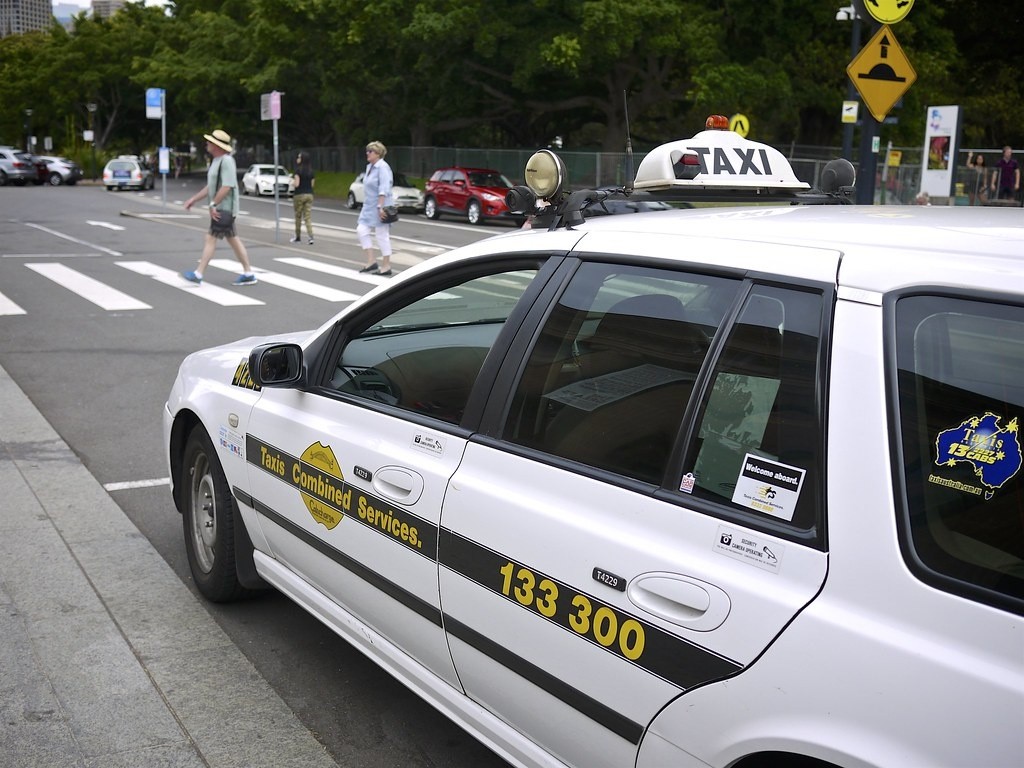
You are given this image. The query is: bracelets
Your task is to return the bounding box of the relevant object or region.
[377,206,382,210]
[209,203,216,208]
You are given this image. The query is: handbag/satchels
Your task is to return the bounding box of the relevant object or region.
[381,205,398,223]
[212,211,233,232]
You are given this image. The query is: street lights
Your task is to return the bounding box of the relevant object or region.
[25,107,34,153]
[85,99,99,182]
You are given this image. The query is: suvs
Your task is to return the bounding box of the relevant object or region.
[423,164,527,228]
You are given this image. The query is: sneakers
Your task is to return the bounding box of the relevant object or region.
[182,271,203,285]
[232,274,257,286]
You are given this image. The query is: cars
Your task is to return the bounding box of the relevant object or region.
[0,145,84,187]
[161,115,1023,768]
[242,163,295,198]
[347,170,425,212]
[102,155,155,192]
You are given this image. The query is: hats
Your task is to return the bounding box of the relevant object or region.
[203,130,233,152]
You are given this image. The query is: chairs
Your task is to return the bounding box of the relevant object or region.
[552,294,715,482]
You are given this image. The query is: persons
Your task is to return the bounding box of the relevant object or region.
[182,130,257,286]
[990,146,1020,200]
[966,152,986,205]
[289,151,316,245]
[356,140,393,277]
[174,154,183,179]
[916,192,930,206]
[34,160,50,185]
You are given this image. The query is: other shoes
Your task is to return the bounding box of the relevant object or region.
[373,269,392,275]
[359,263,378,273]
[307,237,314,245]
[290,236,300,243]
